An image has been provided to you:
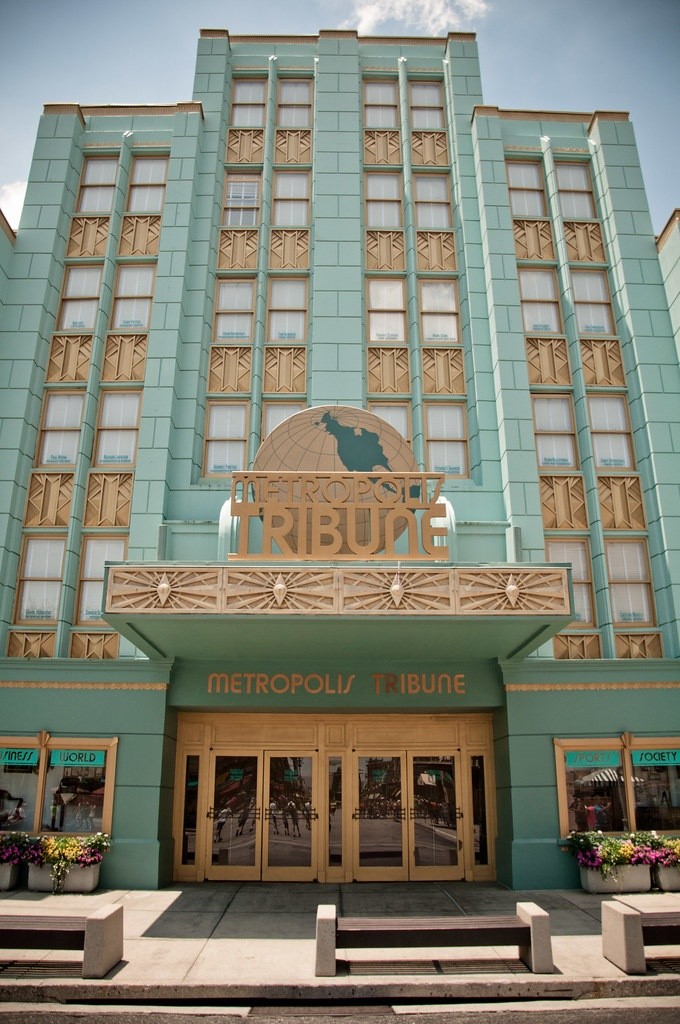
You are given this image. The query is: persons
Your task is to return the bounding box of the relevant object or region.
[214,792,341,843]
[568,794,624,833]
[359,794,457,828]
[74,799,97,831]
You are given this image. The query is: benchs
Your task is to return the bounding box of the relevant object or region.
[600,900,680,976]
[0,904,124,978]
[314,904,554,976]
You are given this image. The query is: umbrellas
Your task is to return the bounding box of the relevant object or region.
[574,766,644,792]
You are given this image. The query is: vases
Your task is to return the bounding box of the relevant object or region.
[580,864,651,893]
[656,863,680,892]
[0,863,16,890]
[27,862,102,893]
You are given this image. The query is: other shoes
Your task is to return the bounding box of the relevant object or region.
[239,830,242,835]
[249,826,253,832]
[235,827,239,837]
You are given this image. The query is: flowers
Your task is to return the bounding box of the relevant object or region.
[562,830,680,871]
[0,829,113,896]
